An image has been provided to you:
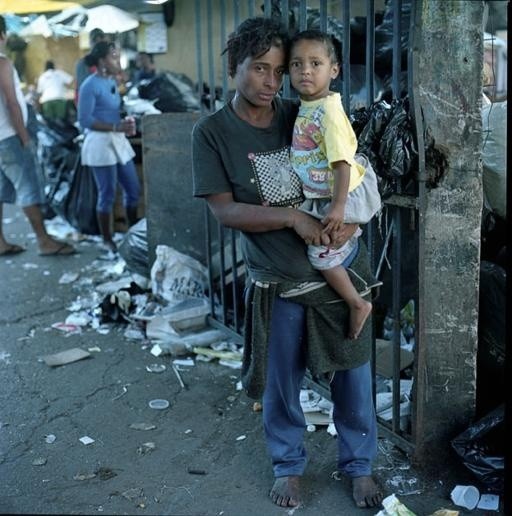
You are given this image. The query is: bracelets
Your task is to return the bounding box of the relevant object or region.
[112,122,117,132]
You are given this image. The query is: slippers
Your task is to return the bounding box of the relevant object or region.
[37,243,76,256]
[0,244,25,256]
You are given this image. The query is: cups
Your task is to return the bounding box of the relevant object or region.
[124,116,136,137]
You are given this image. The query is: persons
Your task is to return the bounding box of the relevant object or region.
[285,30,382,340]
[129,50,158,87]
[36,59,74,123]
[70,26,108,107]
[76,39,143,251]
[190,16,385,507]
[0,14,82,255]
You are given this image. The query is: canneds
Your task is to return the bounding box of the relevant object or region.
[125,117,138,136]
